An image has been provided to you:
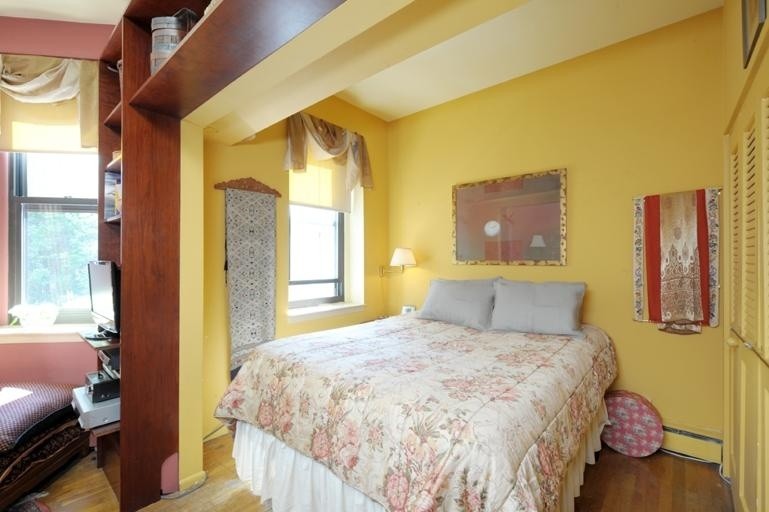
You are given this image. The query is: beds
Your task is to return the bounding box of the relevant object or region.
[227,312,615,512]
[0,384,90,512]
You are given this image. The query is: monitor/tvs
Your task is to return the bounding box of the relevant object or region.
[85,261,121,339]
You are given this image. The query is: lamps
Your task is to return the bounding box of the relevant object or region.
[380,248,418,277]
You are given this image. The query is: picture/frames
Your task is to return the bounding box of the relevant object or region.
[450,166,567,267]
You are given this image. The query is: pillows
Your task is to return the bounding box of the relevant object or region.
[415,277,587,338]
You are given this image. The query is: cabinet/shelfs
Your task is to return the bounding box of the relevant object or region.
[70,0,179,511]
